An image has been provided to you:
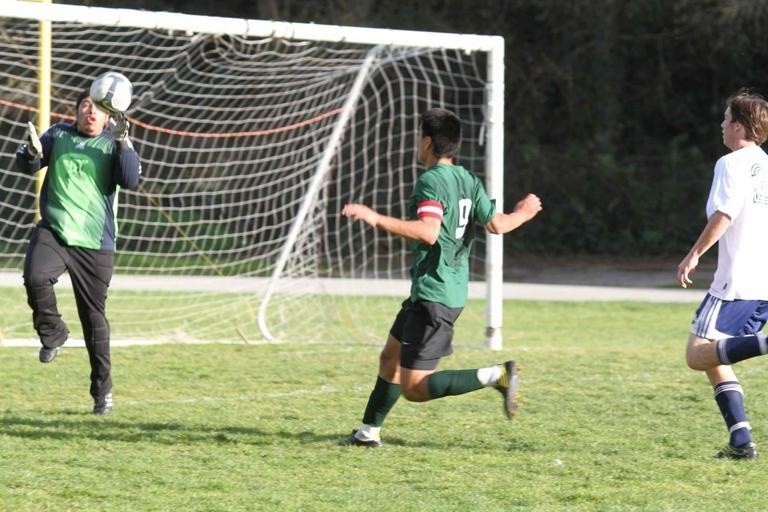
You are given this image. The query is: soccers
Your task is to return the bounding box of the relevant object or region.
[89,70,134,117]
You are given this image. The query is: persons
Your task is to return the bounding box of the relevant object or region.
[333,109,544,449]
[15,89,142,413]
[676,92,768,460]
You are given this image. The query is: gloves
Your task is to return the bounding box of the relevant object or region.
[107,112,131,142]
[23,120,44,159]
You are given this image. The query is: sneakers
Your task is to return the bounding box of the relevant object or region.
[91,393,113,415]
[710,442,759,460]
[38,330,69,363]
[490,358,522,421]
[337,429,383,448]
